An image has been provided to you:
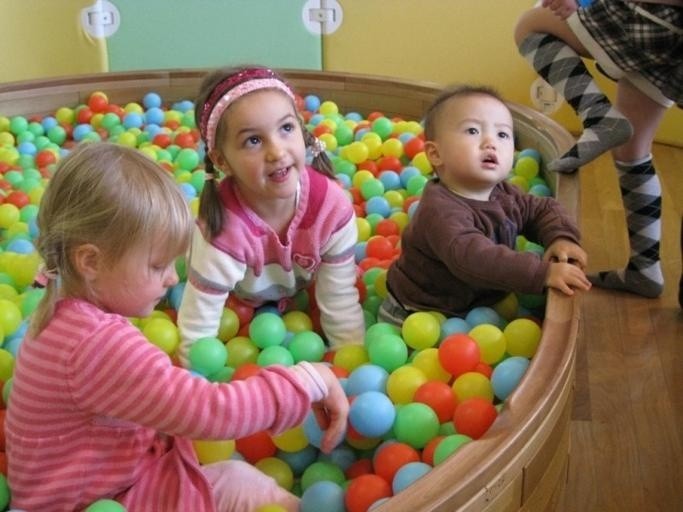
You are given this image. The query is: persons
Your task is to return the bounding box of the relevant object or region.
[5,141,349,510]
[175,62,366,376]
[377,87,592,328]
[514,1,682,299]
